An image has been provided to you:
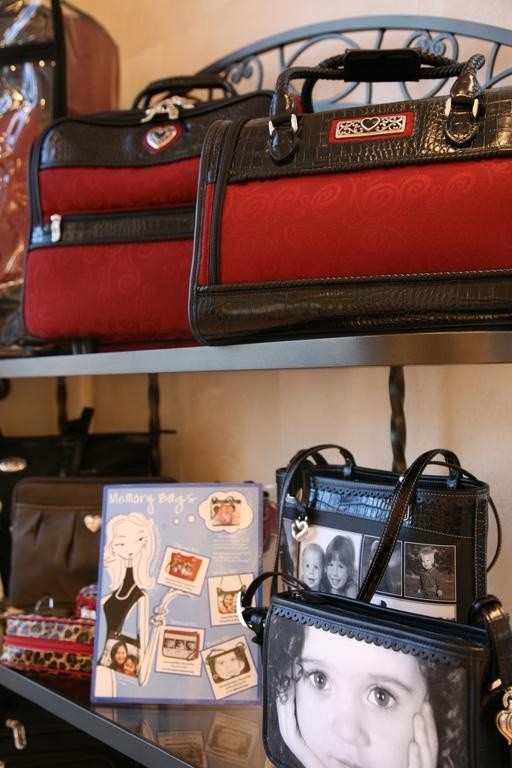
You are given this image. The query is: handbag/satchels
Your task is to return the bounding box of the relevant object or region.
[236,561,512,768]
[188,49,512,342]
[269,443,503,626]
[20,74,296,343]
[0,477,189,610]
[0,597,100,683]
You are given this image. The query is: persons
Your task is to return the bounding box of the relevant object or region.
[263,614,466,767]
[413,548,441,598]
[367,539,393,593]
[298,542,323,592]
[320,533,360,600]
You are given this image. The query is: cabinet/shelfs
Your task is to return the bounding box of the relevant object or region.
[0,330,510,768]
